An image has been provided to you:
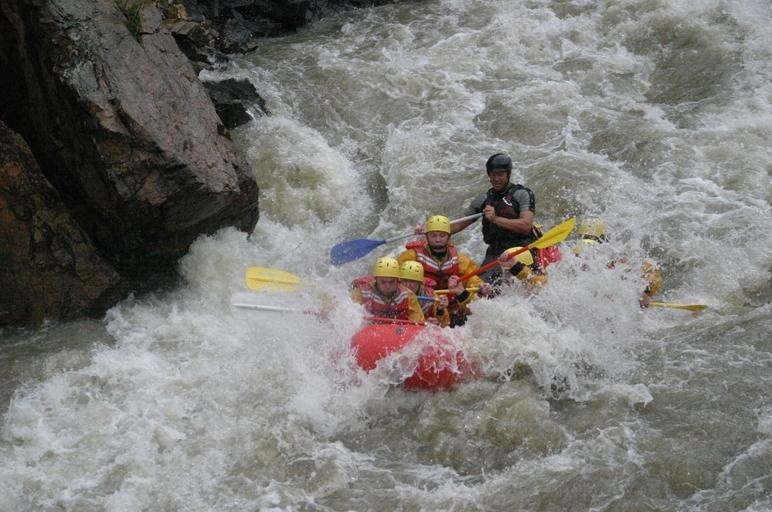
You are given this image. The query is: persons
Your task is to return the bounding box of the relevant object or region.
[413,153,538,284]
[318,214,652,327]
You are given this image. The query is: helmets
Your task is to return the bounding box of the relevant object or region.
[424,214,451,234]
[399,260,424,281]
[502,246,533,269]
[374,257,400,278]
[642,261,662,296]
[573,239,598,255]
[579,218,605,237]
[486,152,512,173]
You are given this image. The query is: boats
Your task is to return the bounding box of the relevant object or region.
[348,236,662,394]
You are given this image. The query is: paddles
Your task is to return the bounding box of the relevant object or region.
[650,302,708,312]
[230,267,440,325]
[457,217,576,282]
[330,213,482,266]
